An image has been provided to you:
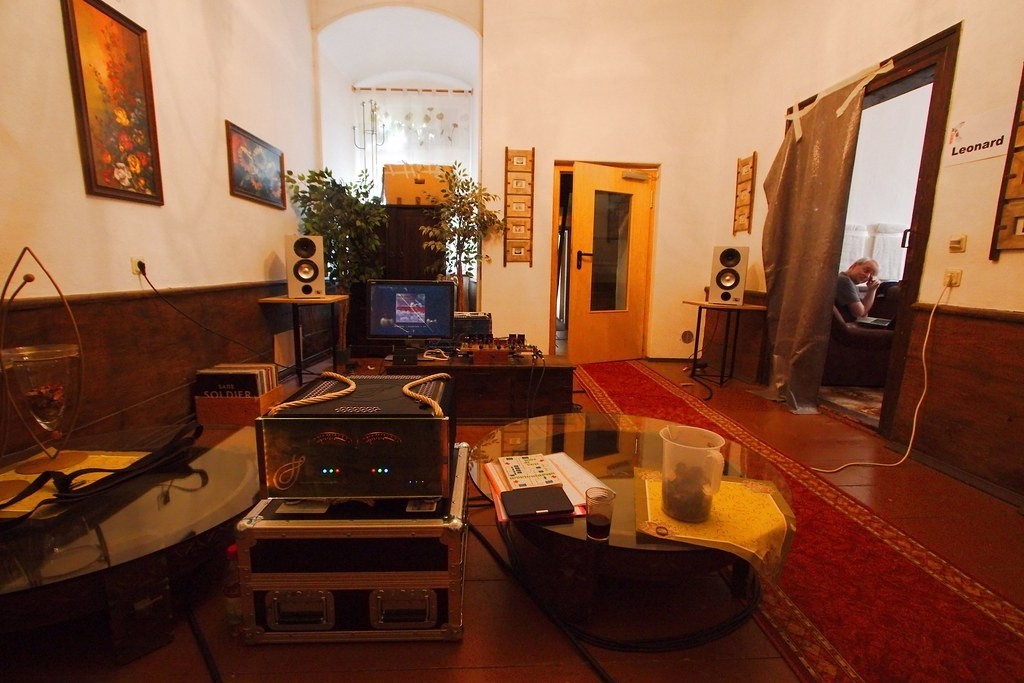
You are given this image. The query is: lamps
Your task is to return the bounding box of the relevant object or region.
[353,98,458,180]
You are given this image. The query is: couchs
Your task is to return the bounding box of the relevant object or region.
[821,302,893,387]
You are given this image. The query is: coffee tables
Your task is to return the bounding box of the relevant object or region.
[468,412,793,648]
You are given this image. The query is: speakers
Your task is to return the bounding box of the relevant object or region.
[285,234,325,300]
[709,246,750,306]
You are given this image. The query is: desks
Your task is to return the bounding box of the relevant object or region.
[257,294,349,384]
[383,350,577,424]
[681,300,766,387]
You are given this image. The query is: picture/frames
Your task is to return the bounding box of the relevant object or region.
[59,0,164,206]
[225,119,287,211]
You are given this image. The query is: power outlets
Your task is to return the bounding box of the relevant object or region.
[943,267,962,287]
[131,256,148,275]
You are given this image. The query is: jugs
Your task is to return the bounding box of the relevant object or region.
[659,425,725,524]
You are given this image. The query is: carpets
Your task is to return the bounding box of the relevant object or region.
[572,357,1024,683]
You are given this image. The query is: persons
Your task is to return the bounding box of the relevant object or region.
[834,257,879,321]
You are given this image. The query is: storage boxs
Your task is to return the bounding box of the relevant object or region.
[195,384,284,425]
[285,167,387,360]
[234,440,470,644]
[384,163,454,206]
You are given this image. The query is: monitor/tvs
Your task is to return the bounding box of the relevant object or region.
[366,279,455,365]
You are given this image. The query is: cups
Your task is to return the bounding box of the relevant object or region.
[585,487,615,540]
[0,344,89,475]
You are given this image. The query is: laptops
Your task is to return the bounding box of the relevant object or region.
[856,314,898,328]
